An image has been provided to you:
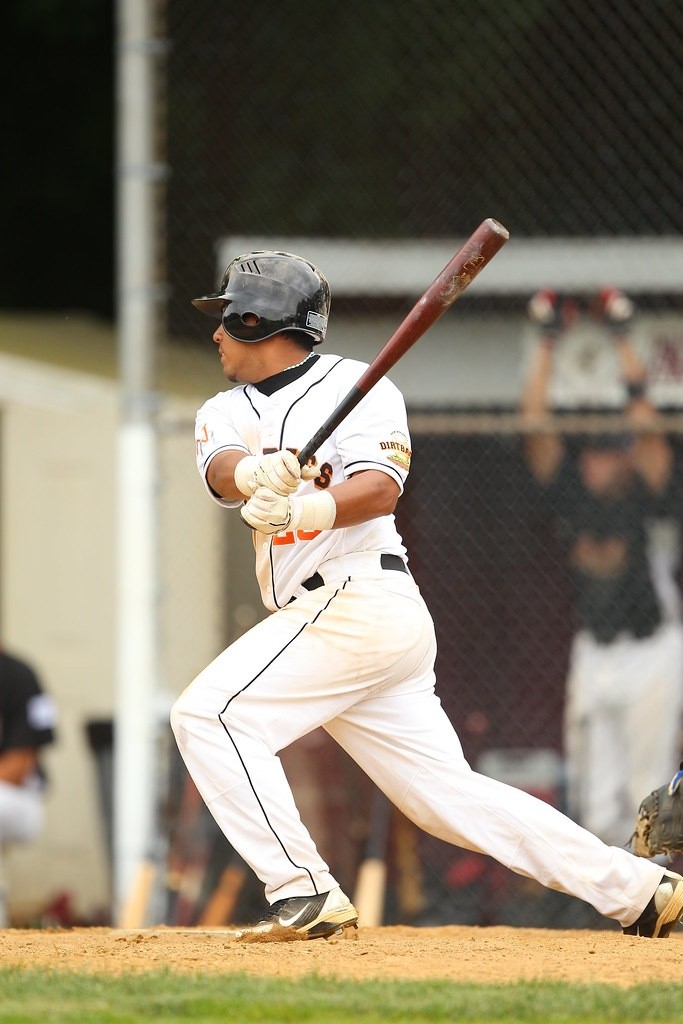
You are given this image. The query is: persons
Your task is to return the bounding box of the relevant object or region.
[0,646,57,844]
[521,283,683,851]
[170,251,683,941]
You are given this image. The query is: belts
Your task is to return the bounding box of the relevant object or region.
[283,553,410,607]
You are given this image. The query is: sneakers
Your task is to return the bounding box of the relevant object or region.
[621,869,683,940]
[235,882,360,941]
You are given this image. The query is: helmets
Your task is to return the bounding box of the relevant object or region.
[190,250,332,347]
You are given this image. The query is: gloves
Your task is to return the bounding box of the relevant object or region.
[234,450,321,497]
[240,485,338,536]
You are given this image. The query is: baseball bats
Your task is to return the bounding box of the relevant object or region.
[236,216,511,532]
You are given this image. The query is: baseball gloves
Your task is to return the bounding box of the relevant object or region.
[632,769,683,859]
